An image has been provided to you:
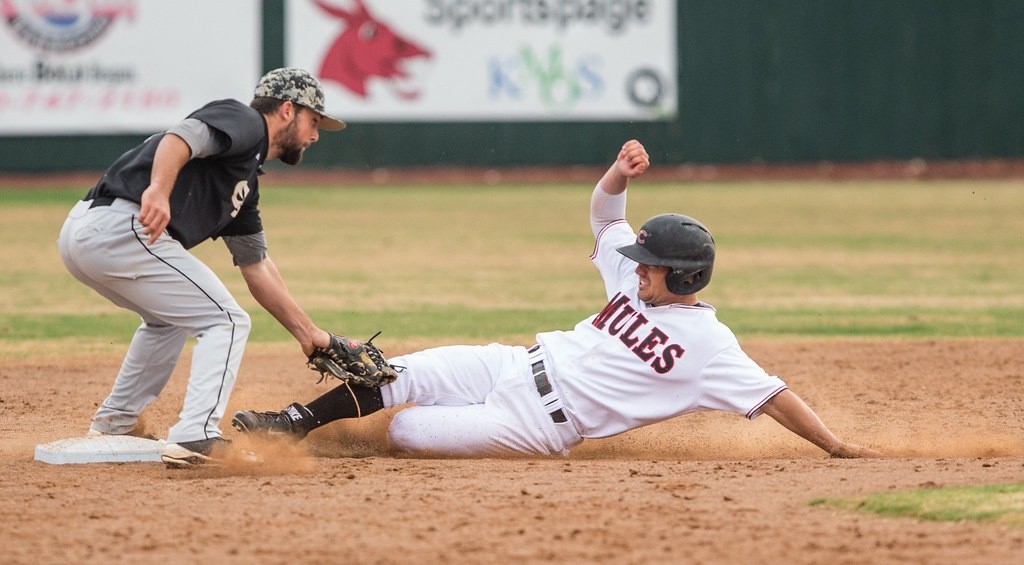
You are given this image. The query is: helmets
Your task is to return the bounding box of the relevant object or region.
[615,213,716,294]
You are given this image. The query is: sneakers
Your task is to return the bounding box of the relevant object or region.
[161,439,271,476]
[232,402,312,453]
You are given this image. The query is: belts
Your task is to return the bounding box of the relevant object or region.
[529,345,568,422]
[89,197,116,210]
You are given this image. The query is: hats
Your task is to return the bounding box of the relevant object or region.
[254,67,346,131]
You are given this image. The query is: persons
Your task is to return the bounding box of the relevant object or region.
[58,66,408,472]
[230,137,882,458]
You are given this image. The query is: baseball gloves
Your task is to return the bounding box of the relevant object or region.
[306,331,398,387]
